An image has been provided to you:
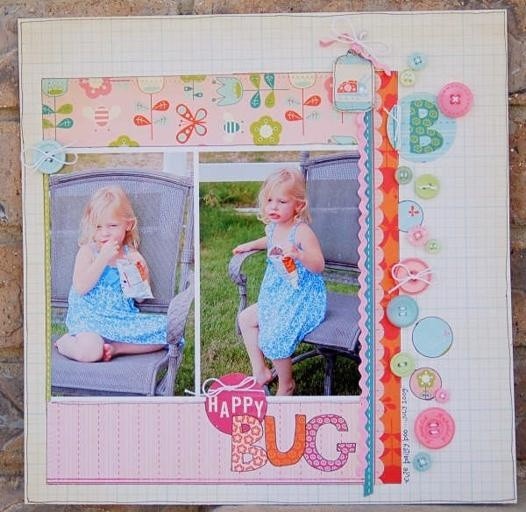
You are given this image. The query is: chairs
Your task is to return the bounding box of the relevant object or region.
[223,147,364,397]
[49,168,196,397]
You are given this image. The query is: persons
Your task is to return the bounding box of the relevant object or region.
[232,167,328,396]
[53,184,185,363]
